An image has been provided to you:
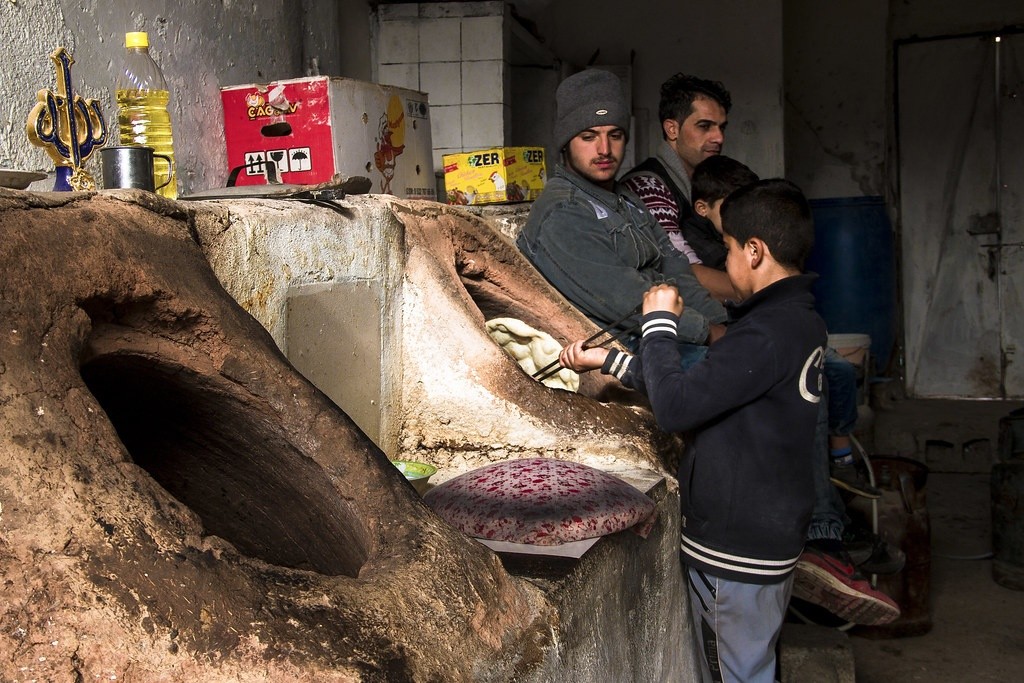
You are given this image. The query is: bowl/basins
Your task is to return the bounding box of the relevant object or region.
[391,460,437,492]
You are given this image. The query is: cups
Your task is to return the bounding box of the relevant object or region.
[100,146,172,192]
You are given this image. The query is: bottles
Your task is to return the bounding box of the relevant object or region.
[115,32,177,201]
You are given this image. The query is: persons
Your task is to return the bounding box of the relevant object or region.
[559,179,827,683]
[517,69,901,631]
[616,73,906,577]
[679,155,882,499]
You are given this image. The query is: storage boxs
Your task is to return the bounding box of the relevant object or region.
[442,145,547,206]
[221,75,437,202]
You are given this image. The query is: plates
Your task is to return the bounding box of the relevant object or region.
[0,170,48,190]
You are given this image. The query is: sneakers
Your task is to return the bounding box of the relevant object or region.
[843,527,905,578]
[830,463,882,499]
[790,547,901,632]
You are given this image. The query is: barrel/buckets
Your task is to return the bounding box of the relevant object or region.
[827,334,871,415]
[805,196,898,377]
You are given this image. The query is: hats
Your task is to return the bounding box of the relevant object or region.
[555,69,630,152]
